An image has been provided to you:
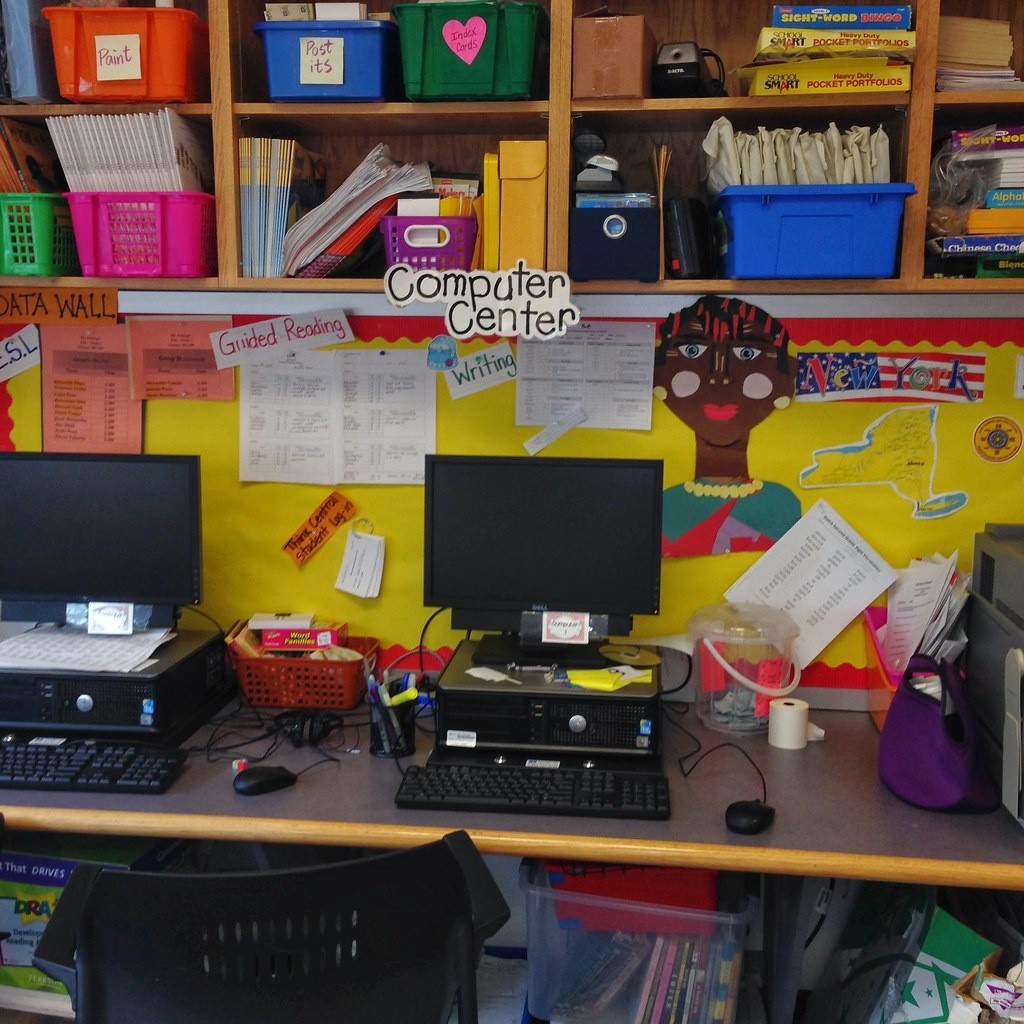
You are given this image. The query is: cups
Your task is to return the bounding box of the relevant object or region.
[368,703,416,759]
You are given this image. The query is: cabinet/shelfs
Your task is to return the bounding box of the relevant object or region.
[0,0,1024,293]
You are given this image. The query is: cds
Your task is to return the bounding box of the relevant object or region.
[599,645,663,666]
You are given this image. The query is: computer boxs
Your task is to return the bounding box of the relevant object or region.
[0,629,229,737]
[436,639,662,751]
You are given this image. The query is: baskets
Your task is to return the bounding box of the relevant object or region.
[383,210,477,271]
[229,637,379,711]
[63,189,213,277]
[0,193,81,276]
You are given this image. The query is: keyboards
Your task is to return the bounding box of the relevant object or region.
[395,764,672,822]
[0,746,191,796]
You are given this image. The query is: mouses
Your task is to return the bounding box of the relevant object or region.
[726,798,775,834]
[232,766,297,796]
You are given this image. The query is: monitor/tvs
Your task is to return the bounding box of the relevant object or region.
[423,454,663,668]
[0,451,203,638]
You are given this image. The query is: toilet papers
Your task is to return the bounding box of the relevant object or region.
[768,697,826,750]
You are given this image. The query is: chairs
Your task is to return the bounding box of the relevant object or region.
[33,828,512,1023]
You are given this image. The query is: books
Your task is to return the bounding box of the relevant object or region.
[550,932,740,1024]
[0,846,129,1020]
[0,107,1024,283]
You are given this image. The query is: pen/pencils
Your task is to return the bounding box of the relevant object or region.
[515,665,556,672]
[368,669,425,754]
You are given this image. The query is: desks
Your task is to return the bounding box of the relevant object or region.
[0,709,1024,888]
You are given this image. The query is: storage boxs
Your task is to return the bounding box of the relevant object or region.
[251,20,400,102]
[391,1,550,100]
[0,191,82,275]
[709,183,919,277]
[500,139,546,271]
[573,4,656,100]
[236,636,381,708]
[381,214,480,279]
[40,6,210,103]
[802,881,1001,1024]
[520,864,751,1023]
[0,836,193,1022]
[541,857,718,936]
[61,190,214,279]
[569,207,659,280]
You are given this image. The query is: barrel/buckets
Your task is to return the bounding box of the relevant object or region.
[684,602,802,735]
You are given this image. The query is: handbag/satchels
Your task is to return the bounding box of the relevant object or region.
[876,653,992,809]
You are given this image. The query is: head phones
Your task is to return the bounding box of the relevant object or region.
[274,706,344,747]
